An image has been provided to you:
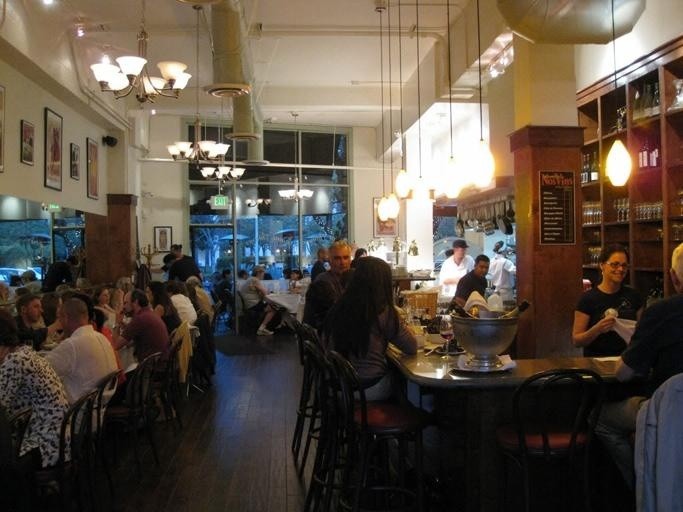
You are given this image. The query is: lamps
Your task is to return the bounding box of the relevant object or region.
[278,112,314,202]
[245,196,272,207]
[396,140,497,201]
[199,94,246,184]
[88,2,191,104]
[608,141,633,187]
[166,4,229,171]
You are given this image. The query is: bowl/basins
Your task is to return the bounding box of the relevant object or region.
[414,269,432,278]
[427,333,444,344]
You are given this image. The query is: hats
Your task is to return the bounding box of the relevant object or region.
[452,241,468,248]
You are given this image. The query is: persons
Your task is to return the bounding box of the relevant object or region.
[585,242,682,495]
[303,239,418,403]
[572,243,646,357]
[211,263,312,336]
[439,239,517,315]
[1,244,213,509]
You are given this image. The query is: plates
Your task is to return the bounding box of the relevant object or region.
[387,263,407,277]
[41,344,57,349]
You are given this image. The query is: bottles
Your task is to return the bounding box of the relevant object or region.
[454,304,475,318]
[580,135,661,185]
[158,229,167,249]
[498,299,531,319]
[632,78,660,124]
[408,318,423,335]
[647,276,665,304]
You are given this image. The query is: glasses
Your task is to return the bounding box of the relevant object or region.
[605,261,631,270]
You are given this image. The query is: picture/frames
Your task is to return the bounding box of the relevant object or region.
[70,142,80,181]
[372,196,398,238]
[42,106,62,192]
[87,136,98,199]
[153,225,172,254]
[0,83,7,172]
[19,119,35,166]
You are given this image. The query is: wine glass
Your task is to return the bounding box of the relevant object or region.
[439,320,454,361]
[586,247,601,266]
[583,198,663,225]
[452,317,519,369]
[665,78,683,112]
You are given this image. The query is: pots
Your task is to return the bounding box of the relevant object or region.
[455,201,515,236]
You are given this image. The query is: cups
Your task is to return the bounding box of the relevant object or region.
[656,224,679,241]
[24,339,33,349]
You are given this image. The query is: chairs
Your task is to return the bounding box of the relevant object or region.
[599,371,682,512]
[2,289,235,509]
[284,312,437,511]
[496,368,605,511]
[237,290,267,335]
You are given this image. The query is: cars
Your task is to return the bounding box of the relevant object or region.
[0,262,44,290]
[433,236,465,271]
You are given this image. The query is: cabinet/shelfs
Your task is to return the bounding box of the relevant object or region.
[576,37,682,310]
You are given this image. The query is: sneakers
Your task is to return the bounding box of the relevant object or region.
[257,328,273,335]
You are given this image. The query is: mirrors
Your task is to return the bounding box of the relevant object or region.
[1,194,108,303]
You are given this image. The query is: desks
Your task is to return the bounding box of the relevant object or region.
[263,291,301,331]
